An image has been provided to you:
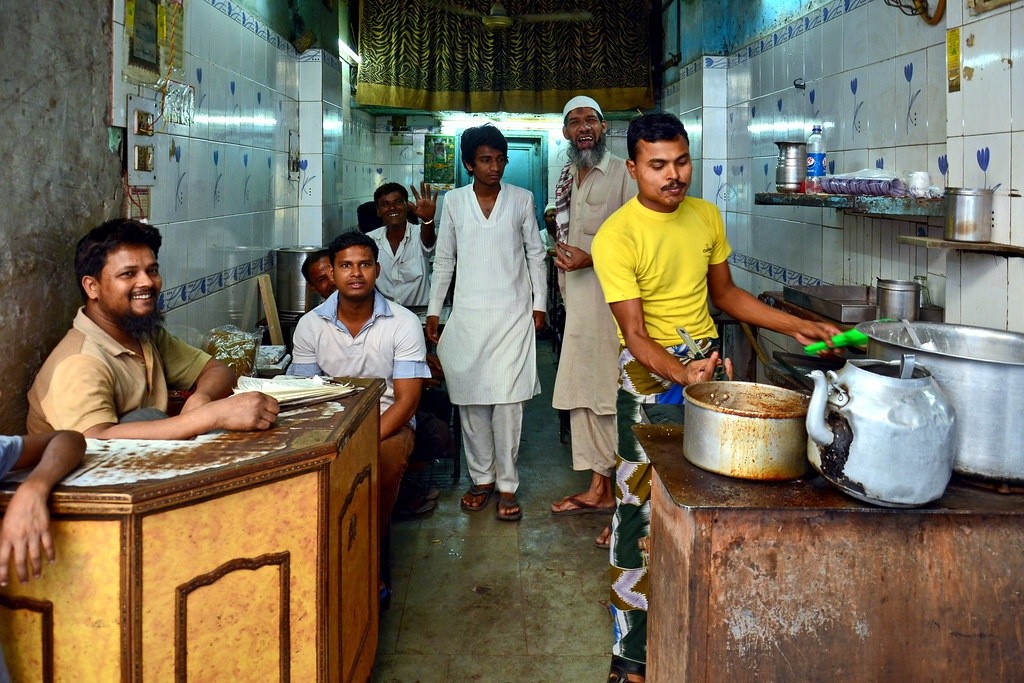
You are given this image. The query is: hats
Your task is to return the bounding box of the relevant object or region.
[562,96,602,125]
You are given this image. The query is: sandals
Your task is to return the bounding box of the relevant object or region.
[379,576,387,600]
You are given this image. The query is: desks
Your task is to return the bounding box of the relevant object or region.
[549,255,559,354]
[256,303,461,484]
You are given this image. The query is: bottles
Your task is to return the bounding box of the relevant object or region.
[774,141,808,194]
[805,125,827,196]
[909,172,930,193]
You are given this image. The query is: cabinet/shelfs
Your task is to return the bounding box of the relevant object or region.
[754,191,946,395]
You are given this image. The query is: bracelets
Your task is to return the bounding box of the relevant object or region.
[422,220,433,224]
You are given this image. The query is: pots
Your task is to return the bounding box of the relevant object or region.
[675,324,812,480]
[855,316,1024,486]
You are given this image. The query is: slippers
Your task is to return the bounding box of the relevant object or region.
[462,482,496,510]
[496,496,521,520]
[554,493,616,515]
[595,523,612,548]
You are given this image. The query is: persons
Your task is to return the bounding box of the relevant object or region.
[552,96,638,548]
[0,429,87,683]
[540,204,557,278]
[287,232,432,541]
[302,181,439,309]
[29,217,279,439]
[427,125,548,522]
[592,113,845,683]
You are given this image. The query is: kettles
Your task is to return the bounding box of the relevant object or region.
[804,352,958,509]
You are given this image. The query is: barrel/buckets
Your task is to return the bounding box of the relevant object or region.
[275,244,330,313]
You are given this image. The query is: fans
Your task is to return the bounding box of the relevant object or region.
[420,0,594,36]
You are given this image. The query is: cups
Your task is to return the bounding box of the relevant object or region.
[876,279,921,322]
[943,186,993,243]
